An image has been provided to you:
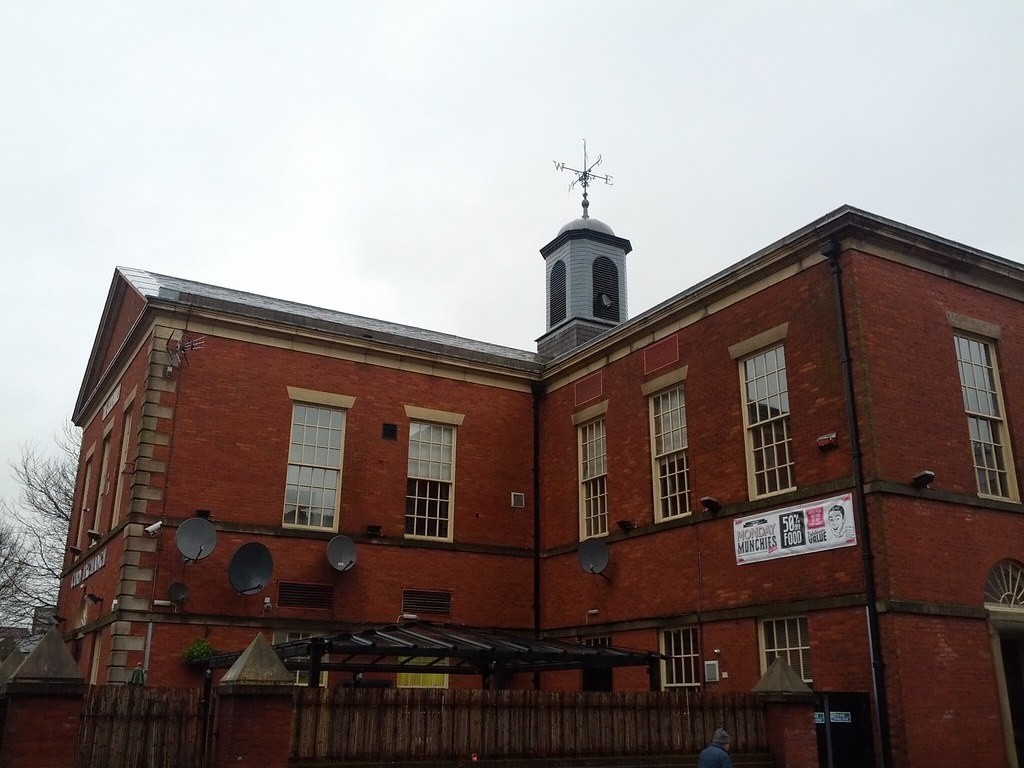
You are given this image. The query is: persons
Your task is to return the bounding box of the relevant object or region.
[697,728,733,768]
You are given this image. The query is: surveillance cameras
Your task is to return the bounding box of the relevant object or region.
[265,603,273,611]
[144,521,163,535]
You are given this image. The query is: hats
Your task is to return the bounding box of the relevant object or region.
[712,728,731,743]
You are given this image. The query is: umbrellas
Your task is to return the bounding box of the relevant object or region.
[127,662,146,685]
